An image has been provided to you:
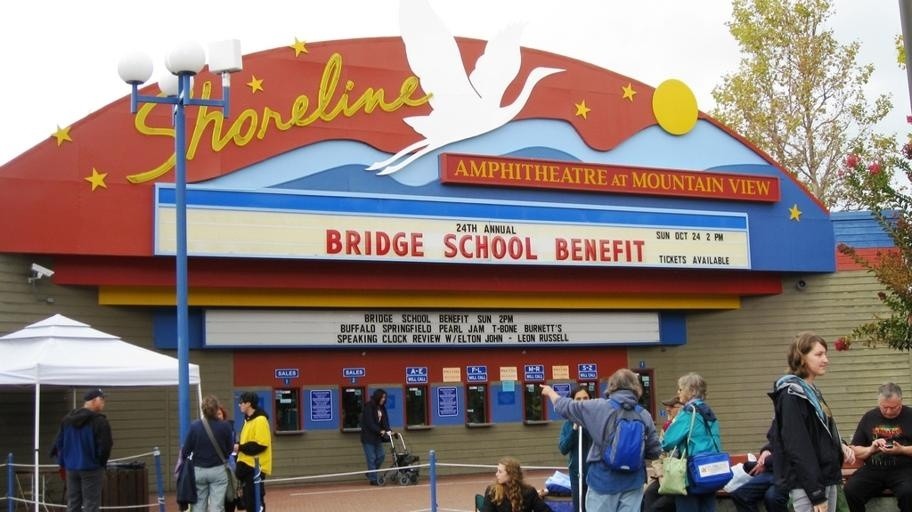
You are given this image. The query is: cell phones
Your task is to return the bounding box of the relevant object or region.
[885,444,893,448]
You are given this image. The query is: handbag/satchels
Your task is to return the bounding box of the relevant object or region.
[687,450,734,495]
[223,465,241,507]
[658,456,690,497]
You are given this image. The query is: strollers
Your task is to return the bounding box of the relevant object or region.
[378,432,422,487]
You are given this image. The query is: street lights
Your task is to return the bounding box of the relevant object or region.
[121,41,245,509]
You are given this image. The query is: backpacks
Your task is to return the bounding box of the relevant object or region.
[597,397,647,476]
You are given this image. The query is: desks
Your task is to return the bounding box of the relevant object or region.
[13,467,62,512]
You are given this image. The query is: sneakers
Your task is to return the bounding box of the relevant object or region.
[366,470,381,487]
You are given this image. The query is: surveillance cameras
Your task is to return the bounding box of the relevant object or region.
[28,263,55,283]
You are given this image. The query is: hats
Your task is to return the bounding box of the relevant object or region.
[84,389,111,400]
[660,394,687,408]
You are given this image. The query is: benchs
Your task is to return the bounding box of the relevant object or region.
[713,445,894,500]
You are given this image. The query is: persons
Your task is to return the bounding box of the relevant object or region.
[766,330,856,512]
[640,396,683,511]
[481,456,553,512]
[559,385,593,512]
[215,403,237,512]
[731,413,791,512]
[843,382,912,511]
[55,388,113,511]
[234,392,273,512]
[361,387,399,485]
[181,394,234,512]
[540,368,662,511]
[660,373,720,511]
